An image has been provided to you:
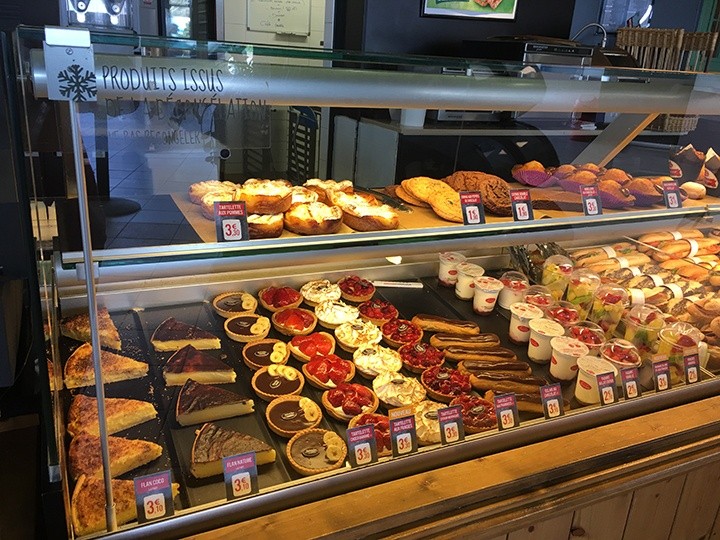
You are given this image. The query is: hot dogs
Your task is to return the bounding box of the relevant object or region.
[569,228,720,371]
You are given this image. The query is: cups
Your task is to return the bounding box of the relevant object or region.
[528,318,565,364]
[522,284,556,311]
[472,276,503,312]
[566,267,602,321]
[652,321,701,386]
[542,253,575,300]
[624,303,665,367]
[399,109,427,129]
[507,303,544,344]
[592,282,628,340]
[455,262,485,299]
[599,337,642,387]
[549,337,590,381]
[574,355,618,405]
[439,252,466,284]
[497,270,530,309]
[543,301,580,328]
[565,321,607,356]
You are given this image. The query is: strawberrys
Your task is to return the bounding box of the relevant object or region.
[503,276,639,364]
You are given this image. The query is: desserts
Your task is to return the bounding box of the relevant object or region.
[60,307,277,537]
[512,160,706,209]
[212,277,498,475]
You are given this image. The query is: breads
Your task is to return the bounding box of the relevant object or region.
[383,169,517,222]
[185,177,400,240]
[410,314,572,415]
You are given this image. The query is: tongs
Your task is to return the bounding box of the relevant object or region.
[510,246,534,276]
